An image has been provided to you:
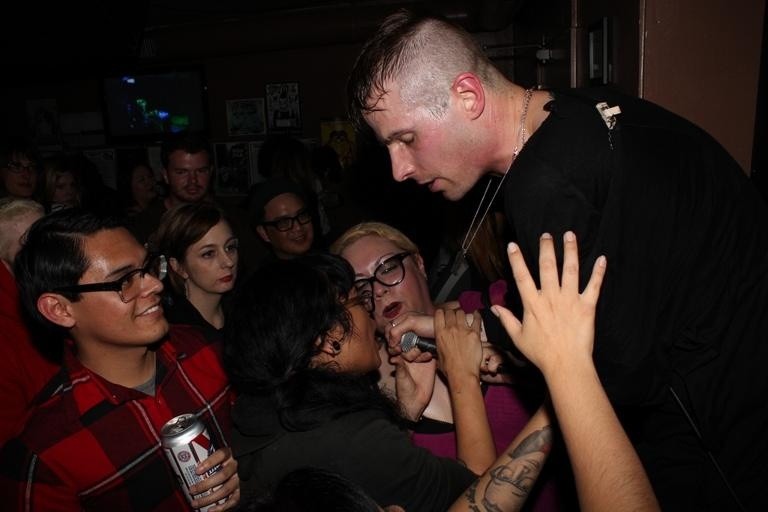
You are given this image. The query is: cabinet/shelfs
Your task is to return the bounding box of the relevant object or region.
[568,0,765,179]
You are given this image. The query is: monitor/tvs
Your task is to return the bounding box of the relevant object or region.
[94,68,212,148]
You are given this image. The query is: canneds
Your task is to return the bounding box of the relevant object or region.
[159,413,232,511]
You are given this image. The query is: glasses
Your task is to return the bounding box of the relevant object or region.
[263,209,313,232]
[76,252,170,305]
[351,249,419,300]
[330,288,376,315]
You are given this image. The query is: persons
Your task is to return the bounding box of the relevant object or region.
[346,9,768,512]
[0,128,661,511]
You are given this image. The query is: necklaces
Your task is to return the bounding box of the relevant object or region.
[453,85,535,274]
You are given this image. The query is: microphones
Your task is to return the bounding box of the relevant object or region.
[399,333,504,376]
